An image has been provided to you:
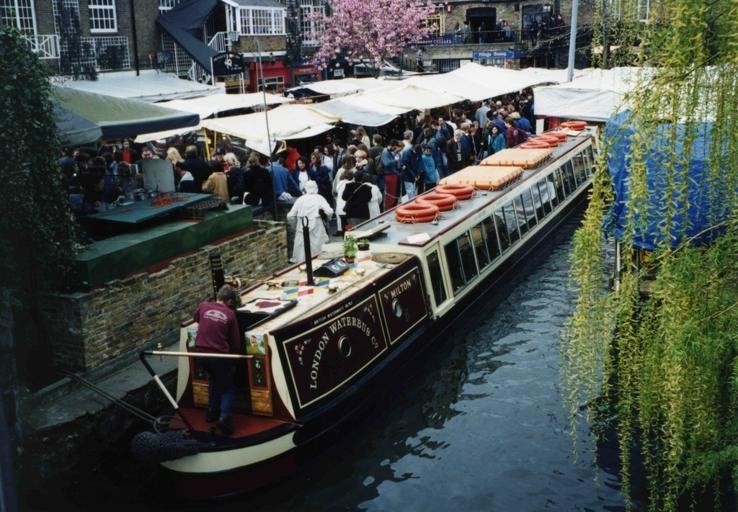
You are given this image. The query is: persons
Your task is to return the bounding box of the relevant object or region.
[452,15,568,44]
[416,47,427,72]
[53,87,549,265]
[192,284,242,434]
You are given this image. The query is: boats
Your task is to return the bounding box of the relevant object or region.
[121,123,604,476]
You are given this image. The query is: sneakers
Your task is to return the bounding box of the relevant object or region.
[206,418,216,423]
[217,417,231,429]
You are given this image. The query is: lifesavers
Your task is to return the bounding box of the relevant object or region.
[394,121,587,222]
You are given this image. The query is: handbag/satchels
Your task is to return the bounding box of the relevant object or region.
[342,194,354,212]
[487,144,494,154]
